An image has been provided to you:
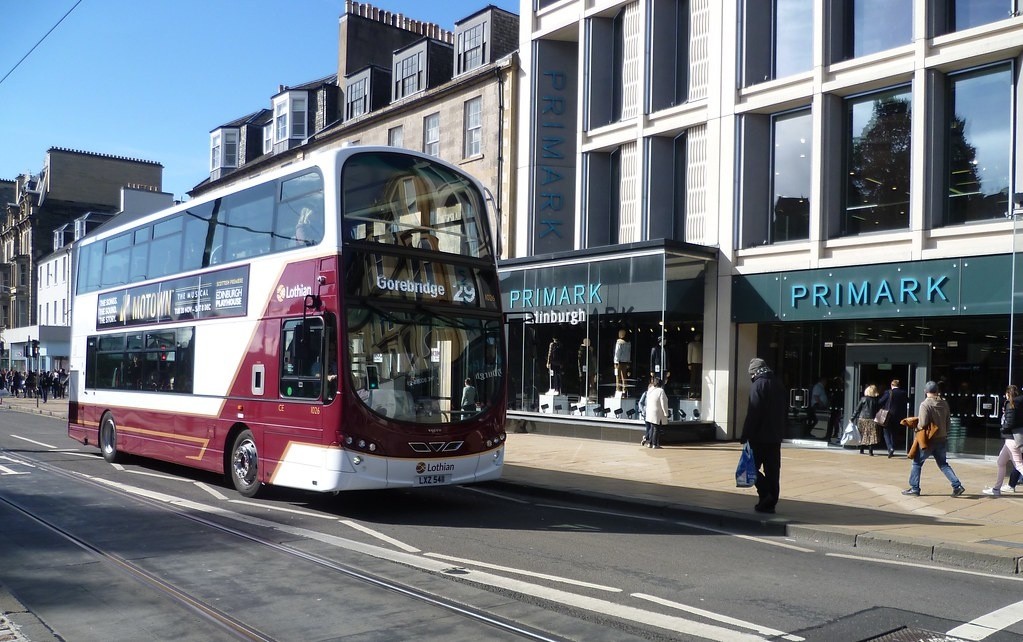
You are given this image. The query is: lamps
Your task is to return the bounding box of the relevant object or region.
[602,408,610,417]
[627,409,636,419]
[693,409,701,422]
[668,410,671,419]
[530,403,537,412]
[614,408,623,418]
[677,409,686,422]
[593,408,602,417]
[569,406,577,416]
[541,404,549,413]
[555,405,563,414]
[579,406,585,416]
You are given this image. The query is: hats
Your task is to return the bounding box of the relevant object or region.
[657,336,666,341]
[748,358,765,373]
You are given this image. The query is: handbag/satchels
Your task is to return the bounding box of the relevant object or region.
[873,390,893,428]
[840,422,862,446]
[735,440,757,488]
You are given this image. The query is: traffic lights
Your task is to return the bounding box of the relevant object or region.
[32,340,40,358]
[0,341,4,356]
[23,345,28,357]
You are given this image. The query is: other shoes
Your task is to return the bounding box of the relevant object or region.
[756,505,775,513]
[641,440,646,445]
[648,444,651,447]
[888,448,894,458]
[653,445,659,448]
[756,498,764,507]
[658,446,661,448]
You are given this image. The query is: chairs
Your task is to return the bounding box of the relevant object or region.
[210,245,222,265]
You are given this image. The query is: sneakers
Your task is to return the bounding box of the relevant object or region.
[1000,484,1015,492]
[982,488,1001,496]
[951,485,965,497]
[902,488,920,496]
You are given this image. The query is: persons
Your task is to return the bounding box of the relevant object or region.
[311,343,338,398]
[547,337,562,392]
[850,385,880,456]
[650,336,671,390]
[739,358,787,514]
[878,380,910,458]
[0,369,68,403]
[461,379,479,418]
[577,338,597,396]
[901,381,965,497]
[687,332,703,398]
[639,379,669,449]
[811,378,828,431]
[614,330,631,394]
[982,385,1023,496]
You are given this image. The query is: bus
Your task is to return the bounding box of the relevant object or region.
[67,143,537,499]
